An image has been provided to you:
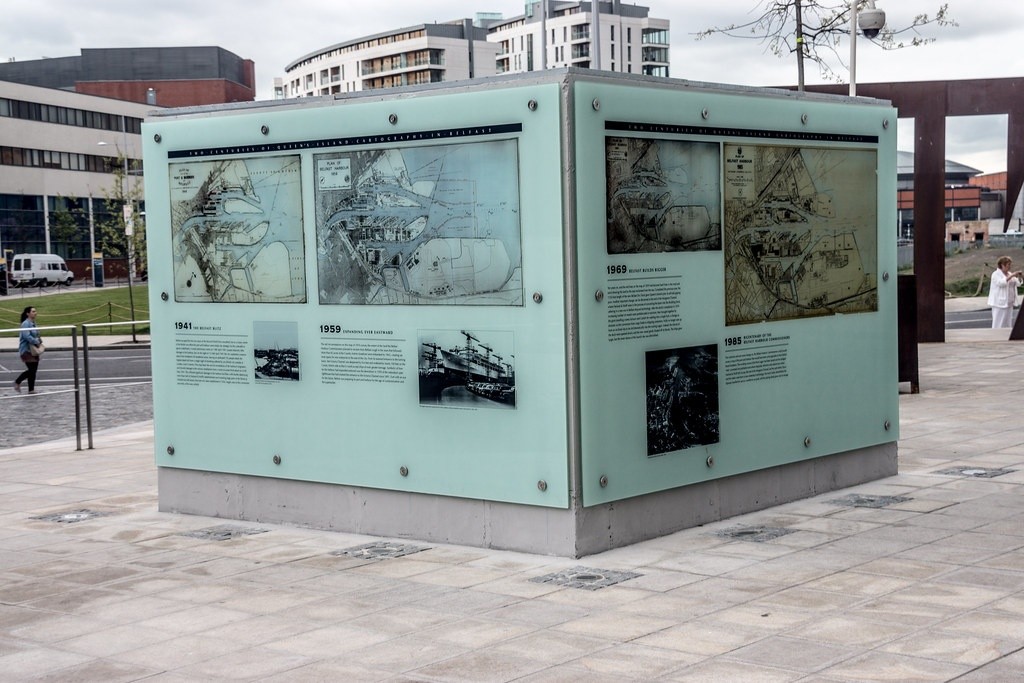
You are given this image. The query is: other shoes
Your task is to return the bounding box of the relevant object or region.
[14,382,21,392]
[28,390,37,394]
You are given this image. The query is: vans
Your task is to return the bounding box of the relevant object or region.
[10,254,74,286]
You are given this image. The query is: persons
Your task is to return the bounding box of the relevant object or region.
[987,256,1023,328]
[15,306,42,394]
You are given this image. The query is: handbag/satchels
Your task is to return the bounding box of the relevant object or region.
[30,336,45,355]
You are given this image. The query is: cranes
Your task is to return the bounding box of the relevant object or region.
[420,330,504,382]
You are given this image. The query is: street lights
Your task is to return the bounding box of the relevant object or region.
[98,142,145,278]
[850,0,885,94]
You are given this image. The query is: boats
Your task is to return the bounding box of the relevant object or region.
[419,346,514,386]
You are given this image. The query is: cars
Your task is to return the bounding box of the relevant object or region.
[466,382,514,403]
